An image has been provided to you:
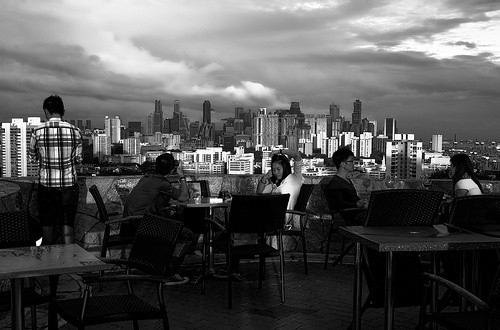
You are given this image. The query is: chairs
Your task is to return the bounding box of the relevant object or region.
[0,179,500,330]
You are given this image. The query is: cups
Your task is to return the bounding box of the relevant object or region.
[221,181,232,199]
[385,177,394,188]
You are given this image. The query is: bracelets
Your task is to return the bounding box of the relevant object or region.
[260,180,266,184]
[180,177,186,182]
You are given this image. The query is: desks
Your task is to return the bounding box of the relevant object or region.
[0,242,106,330]
[170,195,231,284]
[337,223,500,330]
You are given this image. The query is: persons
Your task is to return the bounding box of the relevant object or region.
[448,153,484,200]
[325,147,368,228]
[28,95,83,244]
[120,153,189,286]
[213,154,300,278]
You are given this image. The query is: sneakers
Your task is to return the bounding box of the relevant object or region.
[163,272,189,285]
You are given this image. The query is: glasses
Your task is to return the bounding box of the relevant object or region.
[343,160,353,163]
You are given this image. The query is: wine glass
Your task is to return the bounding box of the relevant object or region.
[422,176,432,190]
